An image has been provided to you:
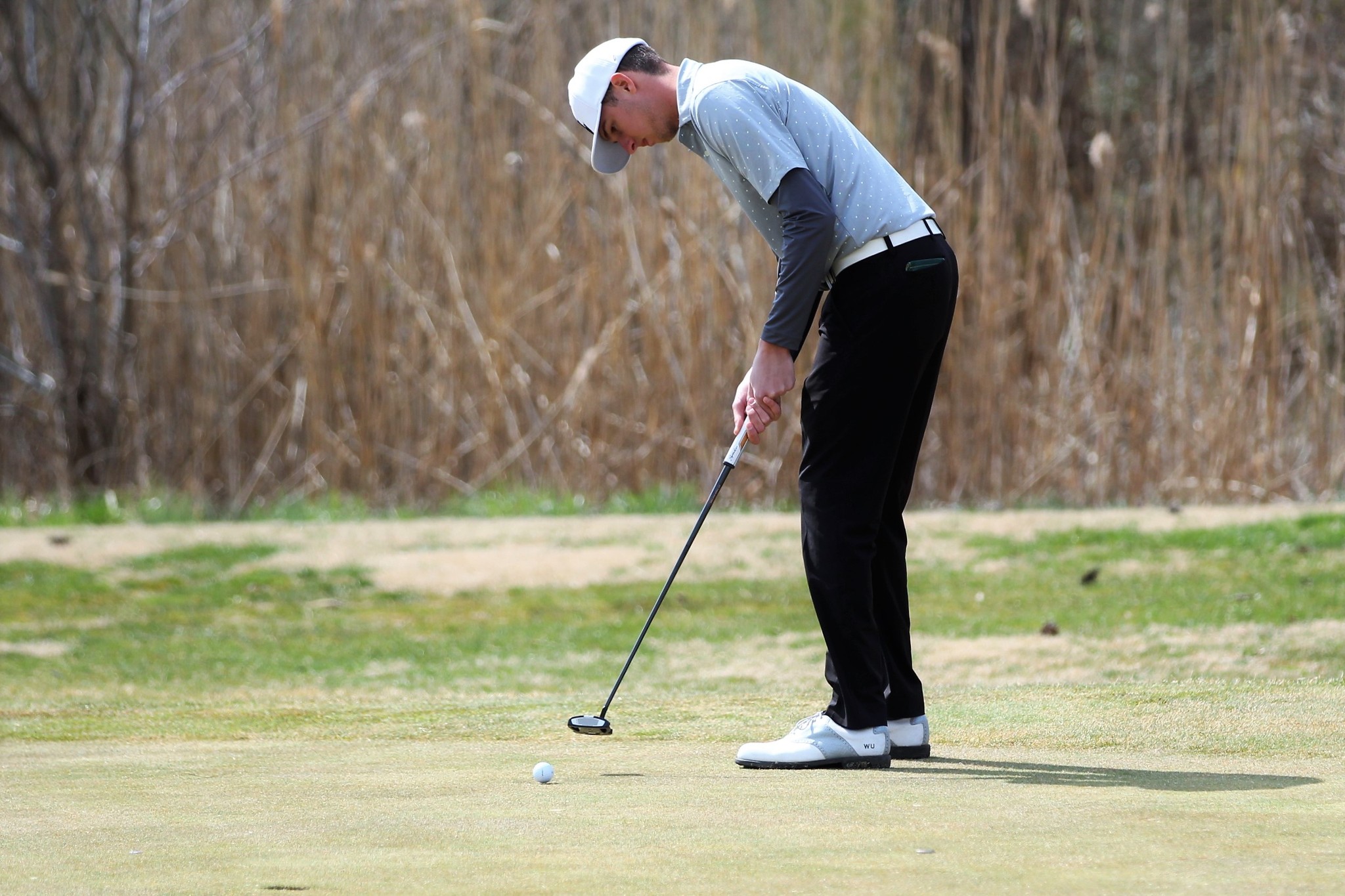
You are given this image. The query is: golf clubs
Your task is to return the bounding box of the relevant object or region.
[566,411,756,736]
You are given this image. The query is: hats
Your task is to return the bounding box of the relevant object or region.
[568,38,648,175]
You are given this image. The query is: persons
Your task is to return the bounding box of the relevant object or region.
[571,38,958,768]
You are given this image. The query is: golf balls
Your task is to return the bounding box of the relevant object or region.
[532,762,554,783]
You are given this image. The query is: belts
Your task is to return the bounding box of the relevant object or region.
[824,218,943,290]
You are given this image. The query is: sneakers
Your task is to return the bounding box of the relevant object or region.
[888,715,930,759]
[735,710,891,769]
